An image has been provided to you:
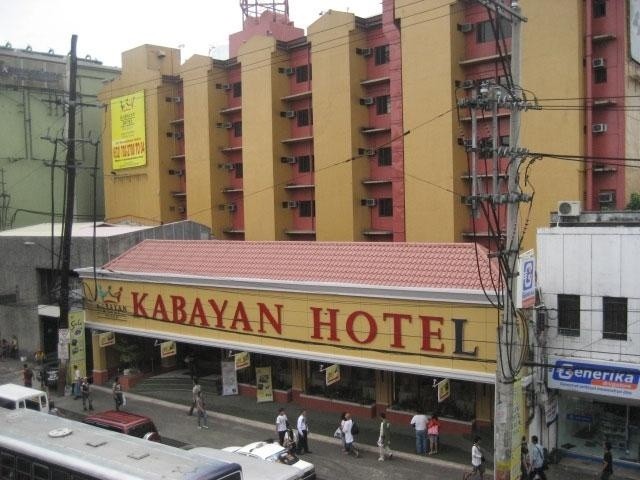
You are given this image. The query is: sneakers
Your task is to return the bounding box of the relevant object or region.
[378,452,392,461]
[197,425,209,428]
[296,450,312,454]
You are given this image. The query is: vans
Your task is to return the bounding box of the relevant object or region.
[181,444,300,480]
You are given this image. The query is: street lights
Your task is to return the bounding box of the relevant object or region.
[24,239,69,397]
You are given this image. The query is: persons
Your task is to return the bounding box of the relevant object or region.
[521,436,529,480]
[411,409,429,454]
[340,412,359,457]
[0,335,129,411]
[186,378,204,416]
[196,391,208,429]
[377,412,393,462]
[427,413,439,455]
[462,436,485,480]
[599,441,613,479]
[276,408,311,454]
[529,436,547,480]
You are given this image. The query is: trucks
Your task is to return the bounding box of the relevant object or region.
[1,383,50,418]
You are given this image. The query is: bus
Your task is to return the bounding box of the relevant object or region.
[0,404,244,480]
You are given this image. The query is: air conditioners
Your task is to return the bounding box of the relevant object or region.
[557,200,581,217]
[593,123,607,133]
[598,190,615,202]
[593,58,607,68]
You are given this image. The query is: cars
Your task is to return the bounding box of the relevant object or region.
[220,440,317,480]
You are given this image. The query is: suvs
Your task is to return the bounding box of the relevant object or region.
[79,409,162,446]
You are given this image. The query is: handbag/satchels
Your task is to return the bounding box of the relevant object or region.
[542,459,550,471]
[351,424,359,435]
